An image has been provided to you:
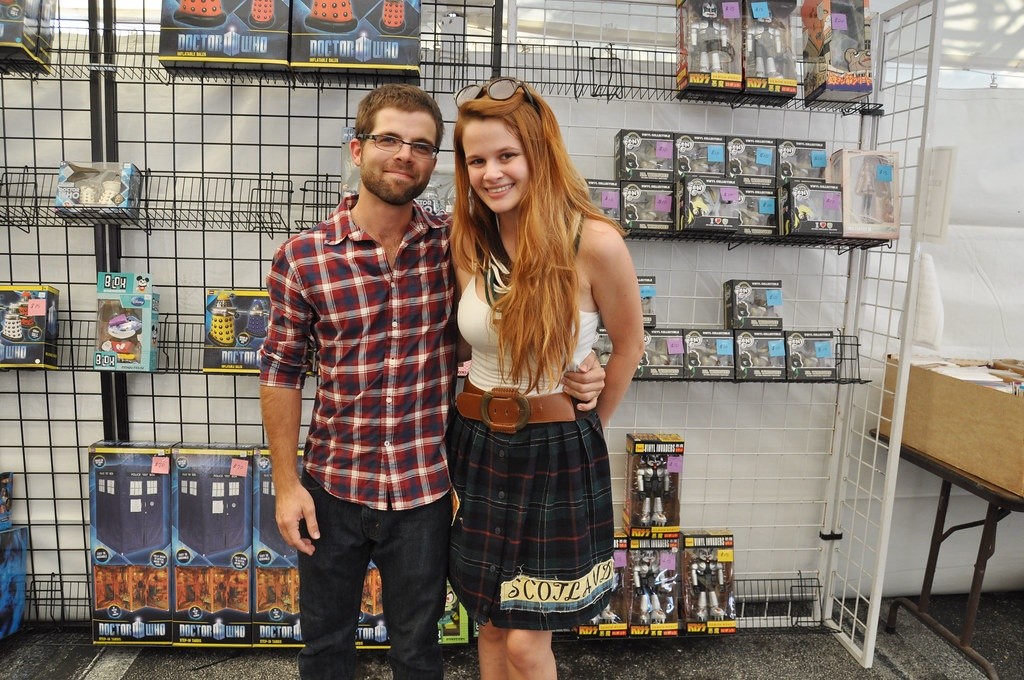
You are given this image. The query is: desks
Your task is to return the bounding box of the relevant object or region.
[869,426,1023,680]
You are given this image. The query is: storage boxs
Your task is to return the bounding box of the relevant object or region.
[878,352,1023,497]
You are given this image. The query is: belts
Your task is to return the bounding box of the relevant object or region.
[457,373,576,432]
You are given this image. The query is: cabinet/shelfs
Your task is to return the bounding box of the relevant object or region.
[0,0,944,667]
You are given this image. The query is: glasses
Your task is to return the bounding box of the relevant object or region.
[452,77,540,115]
[357,133,439,160]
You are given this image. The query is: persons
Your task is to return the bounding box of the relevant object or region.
[629,548,668,624]
[689,545,728,622]
[686,2,731,75]
[450,75,643,680]
[260,84,606,680]
[635,452,671,526]
[744,5,790,83]
